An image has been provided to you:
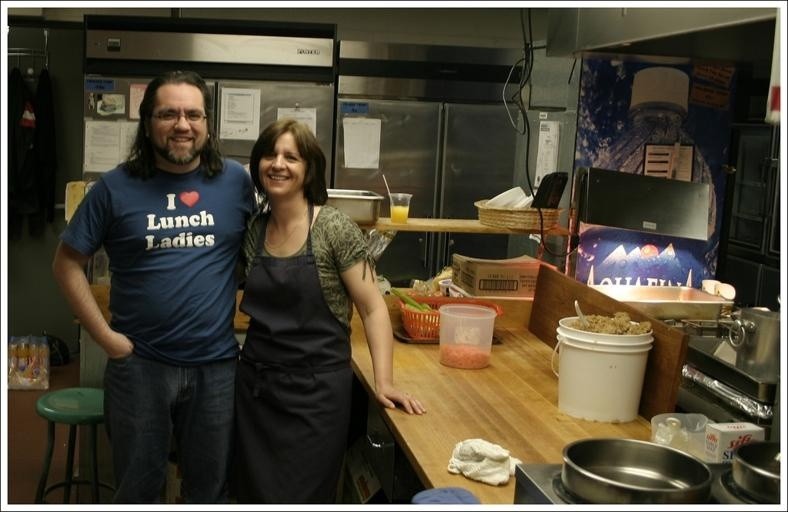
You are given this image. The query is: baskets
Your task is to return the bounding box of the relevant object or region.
[397,297,504,340]
[474,196,566,230]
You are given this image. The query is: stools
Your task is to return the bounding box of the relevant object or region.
[35,386,127,503]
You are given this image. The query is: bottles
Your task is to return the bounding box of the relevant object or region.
[8,333,51,378]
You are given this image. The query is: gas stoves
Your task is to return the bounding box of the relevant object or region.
[510,460,778,505]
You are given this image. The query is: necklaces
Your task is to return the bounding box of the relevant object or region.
[264,220,298,250]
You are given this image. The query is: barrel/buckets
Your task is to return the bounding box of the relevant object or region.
[551,316,655,421]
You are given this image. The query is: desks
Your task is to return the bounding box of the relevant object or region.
[341,282,660,504]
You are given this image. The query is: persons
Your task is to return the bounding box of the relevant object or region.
[95,90,104,113]
[86,91,95,109]
[32,69,55,224]
[46,66,262,506]
[225,116,431,504]
[8,67,38,242]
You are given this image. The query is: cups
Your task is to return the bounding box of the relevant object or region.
[436,301,499,370]
[389,192,414,225]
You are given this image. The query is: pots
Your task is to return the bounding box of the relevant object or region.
[558,436,713,504]
[729,440,780,502]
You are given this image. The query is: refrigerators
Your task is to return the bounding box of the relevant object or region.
[65,7,338,494]
[339,39,527,288]
